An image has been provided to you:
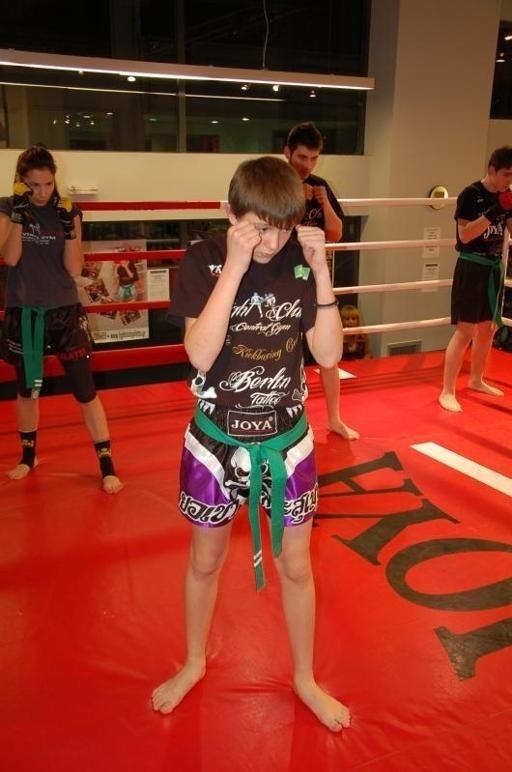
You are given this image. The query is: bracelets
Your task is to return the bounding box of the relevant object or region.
[314,299,341,311]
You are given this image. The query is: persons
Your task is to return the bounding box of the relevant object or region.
[1,145,126,497]
[152,155,351,735]
[284,121,361,441]
[436,146,512,414]
[337,303,372,361]
[114,260,139,304]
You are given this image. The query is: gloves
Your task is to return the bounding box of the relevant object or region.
[55,197,77,240]
[10,183,34,224]
